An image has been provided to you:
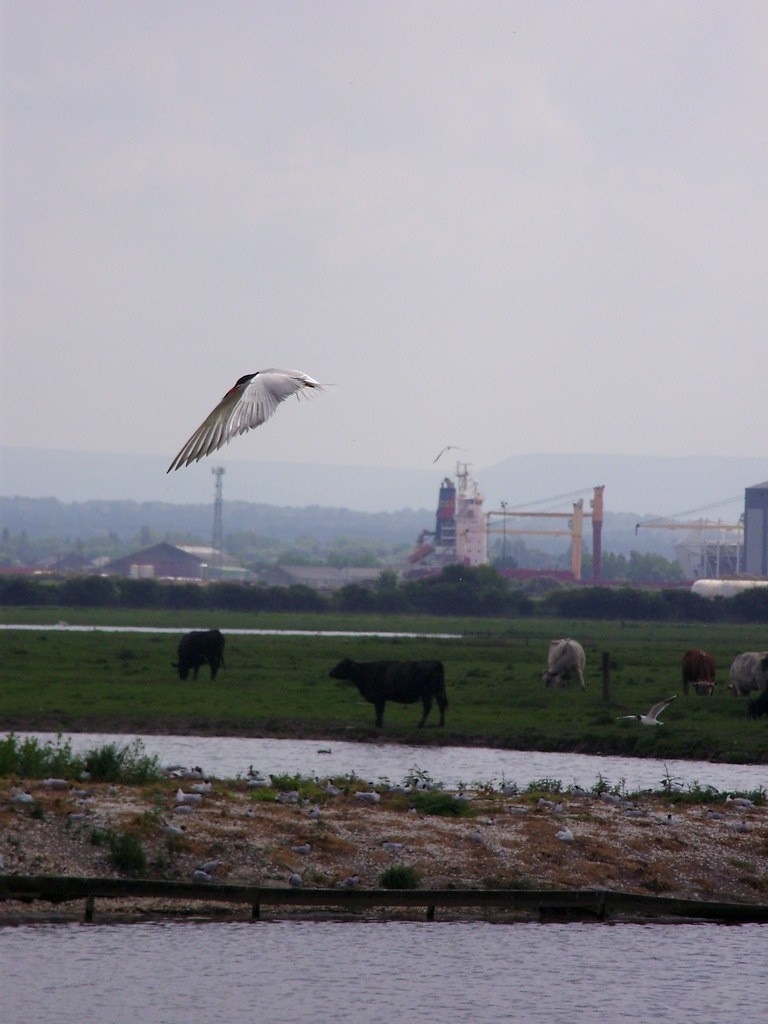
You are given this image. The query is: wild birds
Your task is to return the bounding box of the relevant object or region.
[433,445,467,463]
[165,368,335,474]
[9,763,757,885]
[616,695,677,726]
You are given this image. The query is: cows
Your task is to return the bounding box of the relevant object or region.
[683,648,717,697]
[542,639,586,689]
[728,651,768,719]
[171,631,226,682]
[329,657,448,728]
